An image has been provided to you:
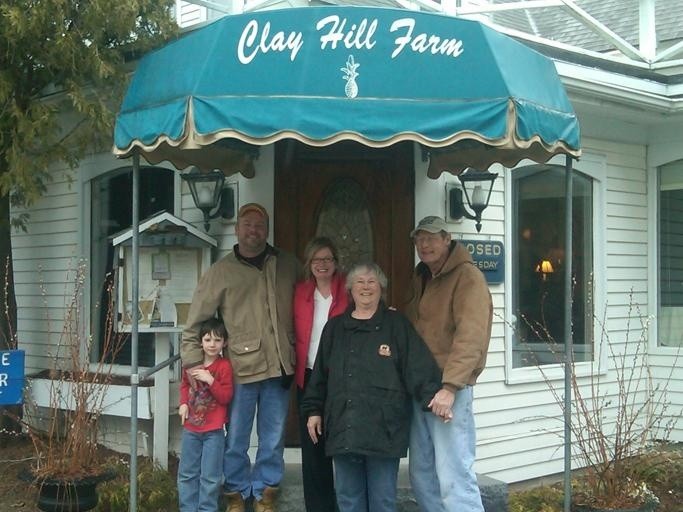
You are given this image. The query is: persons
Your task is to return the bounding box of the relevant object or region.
[290,235,351,509]
[406,215,492,512]
[177,203,300,512]
[174,317,233,509]
[302,261,454,510]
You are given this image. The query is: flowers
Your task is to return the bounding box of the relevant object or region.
[0,254,161,491]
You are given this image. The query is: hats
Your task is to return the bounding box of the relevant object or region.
[236,199,270,219]
[411,216,449,237]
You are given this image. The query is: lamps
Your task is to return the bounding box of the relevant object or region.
[180,167,236,233]
[535,260,553,281]
[447,162,492,233]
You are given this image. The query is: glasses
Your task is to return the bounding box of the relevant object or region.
[311,255,337,266]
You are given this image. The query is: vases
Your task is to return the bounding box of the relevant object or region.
[14,465,118,510]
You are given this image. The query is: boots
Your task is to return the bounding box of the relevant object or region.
[253,486,280,511]
[223,491,247,511]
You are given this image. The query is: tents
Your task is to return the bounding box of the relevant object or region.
[110,2,583,511]
